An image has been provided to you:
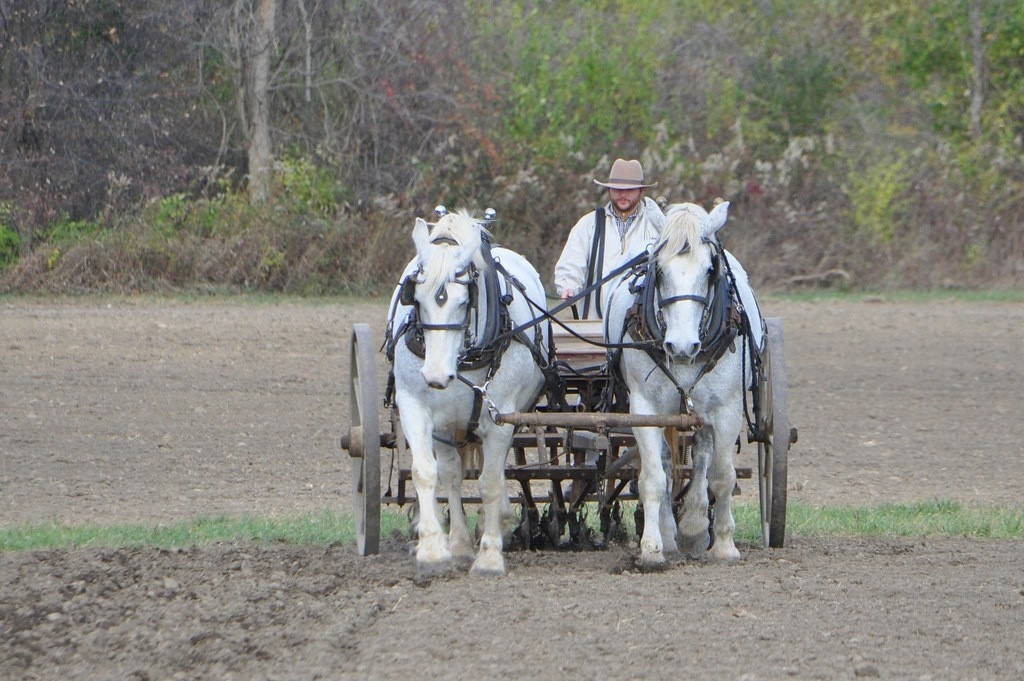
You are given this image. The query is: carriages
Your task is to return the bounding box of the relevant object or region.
[340,201,798,578]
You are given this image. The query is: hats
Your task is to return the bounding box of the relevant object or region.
[594,158,658,189]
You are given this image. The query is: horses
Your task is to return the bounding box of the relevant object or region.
[602,196,766,572]
[386,208,549,578]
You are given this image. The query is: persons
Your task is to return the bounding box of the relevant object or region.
[554,158,665,502]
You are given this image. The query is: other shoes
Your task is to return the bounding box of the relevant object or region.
[630,480,639,495]
[564,481,600,497]
